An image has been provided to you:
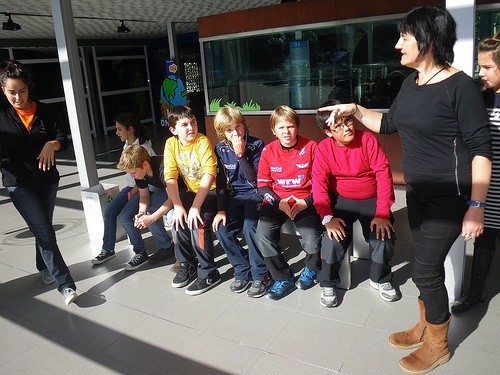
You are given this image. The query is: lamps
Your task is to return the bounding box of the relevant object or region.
[117,21,131,33]
[2,15,22,31]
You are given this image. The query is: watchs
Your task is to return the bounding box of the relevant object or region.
[322,215,333,225]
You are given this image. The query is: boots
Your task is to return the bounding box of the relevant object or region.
[398,314,451,375]
[389,296,425,349]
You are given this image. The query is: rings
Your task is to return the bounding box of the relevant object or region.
[465,234,471,239]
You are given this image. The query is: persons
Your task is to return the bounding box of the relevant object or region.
[214,106,272,298]
[164,105,222,296]
[0,60,77,306]
[256,105,322,301]
[91,110,157,269]
[116,145,187,265]
[311,99,399,308]
[317,5,492,373]
[452,29,500,315]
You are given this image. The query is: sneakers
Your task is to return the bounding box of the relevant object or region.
[171,262,180,273]
[172,263,197,288]
[267,276,296,301]
[321,287,338,307]
[149,242,175,263]
[369,280,399,302]
[185,271,221,295]
[91,248,116,264]
[39,268,55,285]
[295,267,317,290]
[230,279,251,293]
[247,277,272,298]
[63,288,77,305]
[125,254,149,271]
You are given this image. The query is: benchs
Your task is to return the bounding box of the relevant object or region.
[281,220,370,290]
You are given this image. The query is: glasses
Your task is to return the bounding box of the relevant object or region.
[330,118,353,133]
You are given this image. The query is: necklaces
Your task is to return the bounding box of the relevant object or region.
[416,65,448,86]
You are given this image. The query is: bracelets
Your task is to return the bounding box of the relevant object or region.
[467,200,486,207]
[351,103,357,116]
